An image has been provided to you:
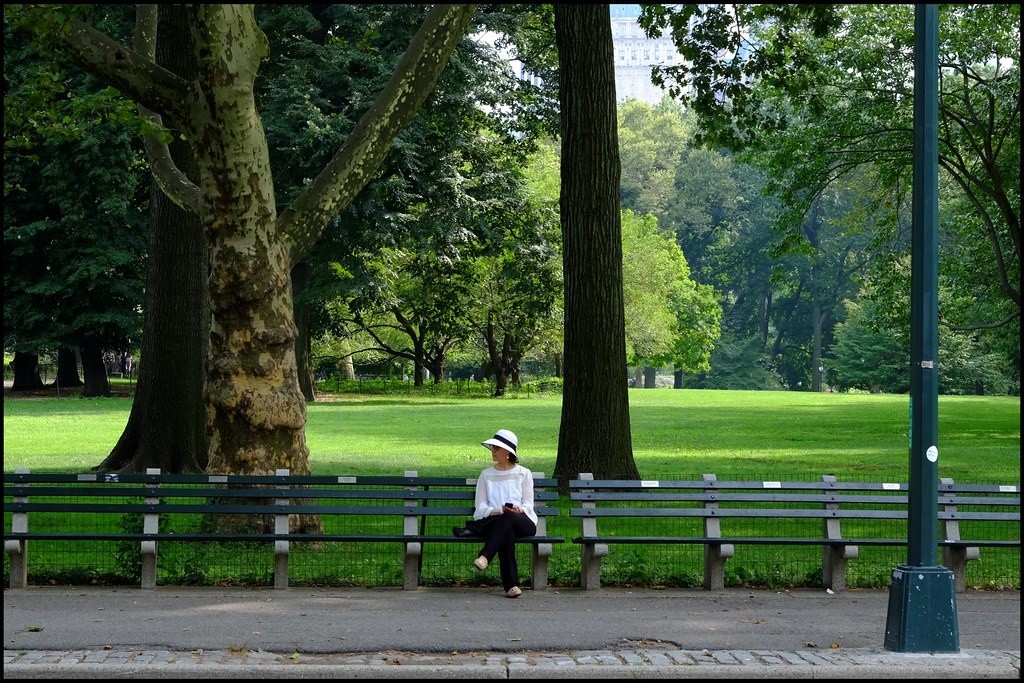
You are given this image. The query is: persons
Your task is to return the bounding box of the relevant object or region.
[472,429,539,597]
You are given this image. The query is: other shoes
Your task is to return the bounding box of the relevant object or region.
[507,586,522,597]
[473,559,487,571]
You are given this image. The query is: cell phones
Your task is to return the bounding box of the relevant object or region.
[505,502,513,509]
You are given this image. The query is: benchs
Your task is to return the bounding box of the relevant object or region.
[568,474,1020,592]
[4,468,565,591]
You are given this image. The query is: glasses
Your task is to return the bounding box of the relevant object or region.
[492,445,501,450]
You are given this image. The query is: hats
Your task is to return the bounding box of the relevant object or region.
[481,429,519,463]
